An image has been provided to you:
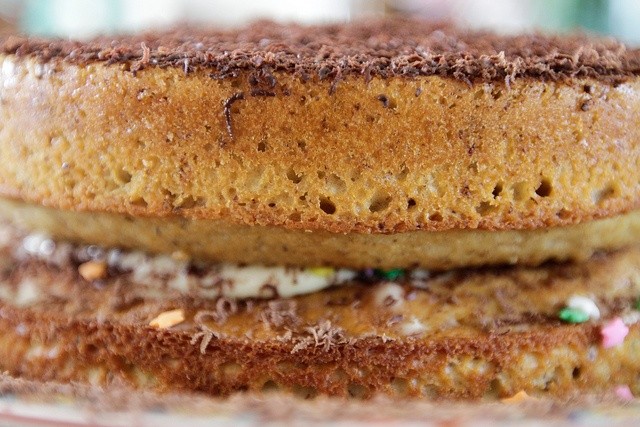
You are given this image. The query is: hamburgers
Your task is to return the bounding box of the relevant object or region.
[0,22,640,424]
